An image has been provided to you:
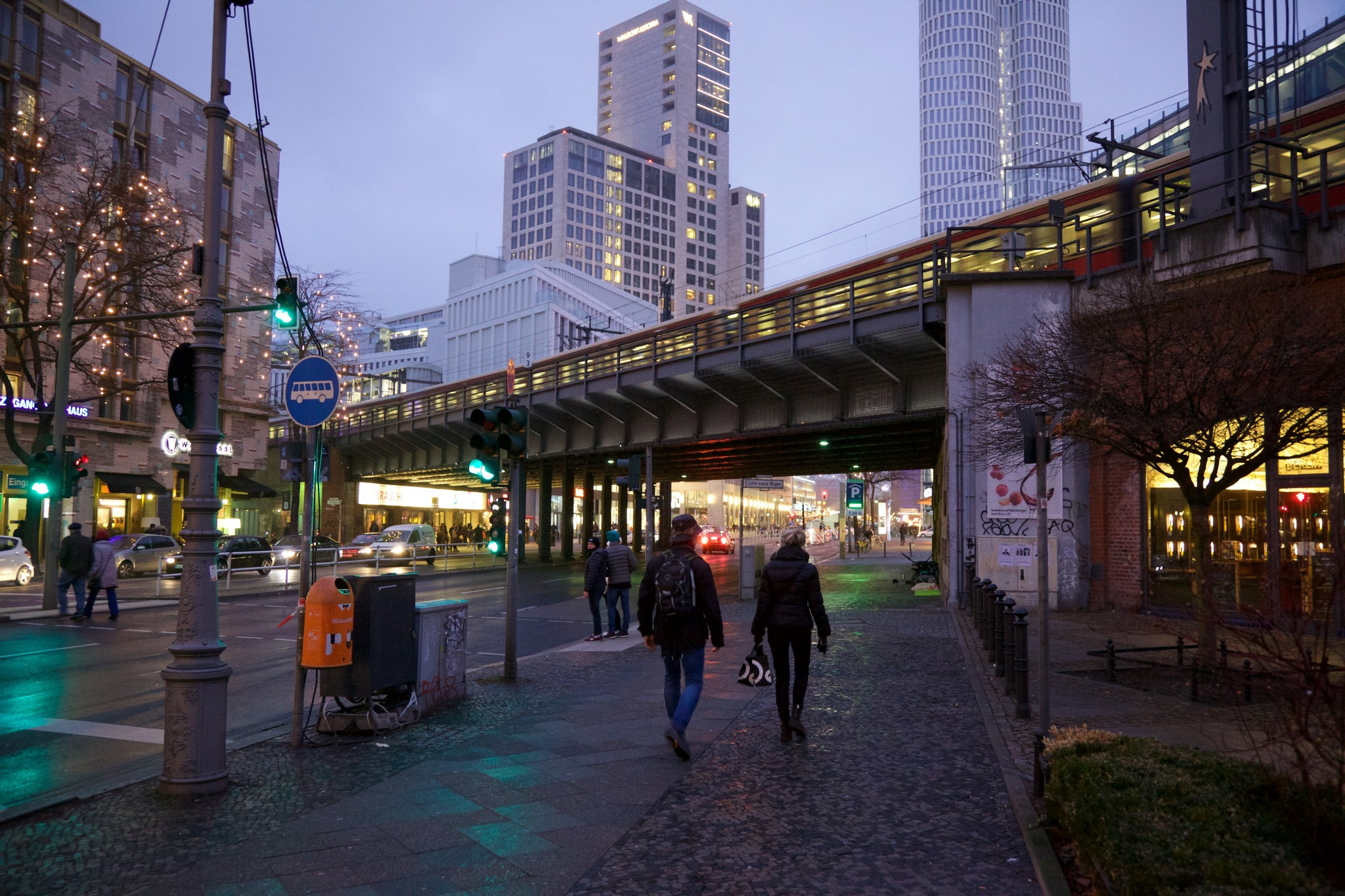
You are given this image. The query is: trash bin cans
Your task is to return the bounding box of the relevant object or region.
[301,575,354,669]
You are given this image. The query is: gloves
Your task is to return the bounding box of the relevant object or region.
[816,637,828,655]
[754,632,764,643]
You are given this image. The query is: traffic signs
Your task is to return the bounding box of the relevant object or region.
[846,478,865,516]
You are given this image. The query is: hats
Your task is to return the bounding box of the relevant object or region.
[606,531,620,542]
[96,531,110,540]
[68,522,82,530]
[588,536,600,549]
[670,514,703,542]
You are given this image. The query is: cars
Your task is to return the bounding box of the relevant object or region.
[341,532,382,564]
[825,526,854,536]
[917,528,933,538]
[702,531,735,555]
[166,536,275,579]
[711,526,731,536]
[698,524,714,536]
[272,532,343,569]
[593,528,659,545]
[0,535,34,586]
[109,534,182,579]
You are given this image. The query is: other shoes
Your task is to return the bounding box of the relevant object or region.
[83,609,91,618]
[70,616,83,620]
[664,725,692,760]
[780,731,792,742]
[788,721,807,738]
[56,614,66,619]
[108,616,118,619]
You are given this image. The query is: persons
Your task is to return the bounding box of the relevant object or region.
[826,526,831,531]
[551,522,558,535]
[283,522,291,536]
[819,524,822,531]
[144,523,168,536]
[635,514,725,761]
[751,529,832,742]
[865,528,873,544]
[1003,549,1030,556]
[583,530,638,641]
[436,522,490,553]
[899,524,932,546]
[525,522,538,544]
[369,520,379,533]
[731,523,781,535]
[13,519,26,541]
[611,521,618,530]
[835,525,839,531]
[56,522,119,621]
[593,522,598,533]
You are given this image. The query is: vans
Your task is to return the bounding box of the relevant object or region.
[361,524,437,567]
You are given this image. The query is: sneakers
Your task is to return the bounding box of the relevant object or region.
[604,631,616,639]
[584,633,604,642]
[615,631,629,638]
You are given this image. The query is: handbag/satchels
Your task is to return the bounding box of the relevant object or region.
[87,578,101,591]
[737,641,773,687]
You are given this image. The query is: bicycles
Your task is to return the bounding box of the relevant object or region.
[851,537,870,553]
[875,534,883,547]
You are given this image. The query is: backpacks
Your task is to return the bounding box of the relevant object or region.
[654,549,702,622]
[452,529,458,538]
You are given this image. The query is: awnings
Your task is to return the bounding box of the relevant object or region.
[220,476,278,499]
[172,462,226,482]
[95,472,167,494]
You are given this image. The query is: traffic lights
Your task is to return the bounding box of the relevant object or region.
[32,452,54,498]
[65,452,88,497]
[616,457,641,491]
[489,498,506,554]
[468,407,503,484]
[497,405,529,459]
[822,491,826,500]
[276,274,301,330]
